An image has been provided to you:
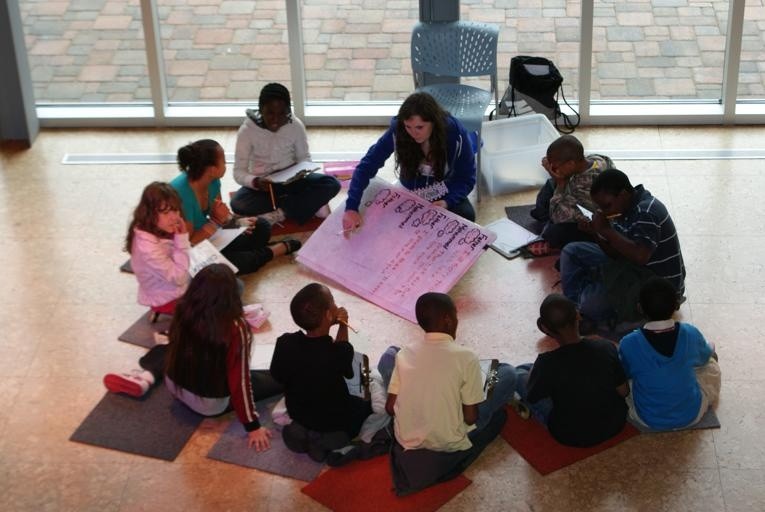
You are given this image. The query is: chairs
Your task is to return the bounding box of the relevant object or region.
[411,21,499,201]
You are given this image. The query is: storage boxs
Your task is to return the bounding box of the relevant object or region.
[479,113,561,196]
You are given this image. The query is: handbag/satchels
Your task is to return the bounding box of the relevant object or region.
[508,54,564,109]
[497,85,562,121]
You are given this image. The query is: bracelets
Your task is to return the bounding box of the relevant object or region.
[230,214,243,225]
[201,216,224,236]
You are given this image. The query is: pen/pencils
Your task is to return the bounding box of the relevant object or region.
[606,213,623,219]
[341,320,358,334]
[338,224,360,236]
[269,183,277,209]
[206,215,220,229]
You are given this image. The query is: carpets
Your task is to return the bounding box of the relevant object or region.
[206,395,326,482]
[120,259,134,274]
[230,192,330,236]
[626,403,721,433]
[506,204,561,259]
[119,309,175,348]
[70,378,205,461]
[300,451,473,512]
[496,405,639,475]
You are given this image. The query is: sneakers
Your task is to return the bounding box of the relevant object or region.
[102,371,151,399]
[258,210,283,226]
[313,203,332,219]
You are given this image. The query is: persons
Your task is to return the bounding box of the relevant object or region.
[339,91,476,239]
[560,168,687,335]
[270,283,371,462]
[521,135,616,257]
[617,283,721,430]
[507,294,630,449]
[231,83,341,226]
[377,292,517,498]
[168,140,301,275]
[104,262,273,453]
[125,182,244,321]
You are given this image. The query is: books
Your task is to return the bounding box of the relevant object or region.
[481,217,545,260]
[478,358,492,387]
[342,352,367,401]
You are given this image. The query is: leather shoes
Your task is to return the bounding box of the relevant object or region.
[264,238,302,256]
